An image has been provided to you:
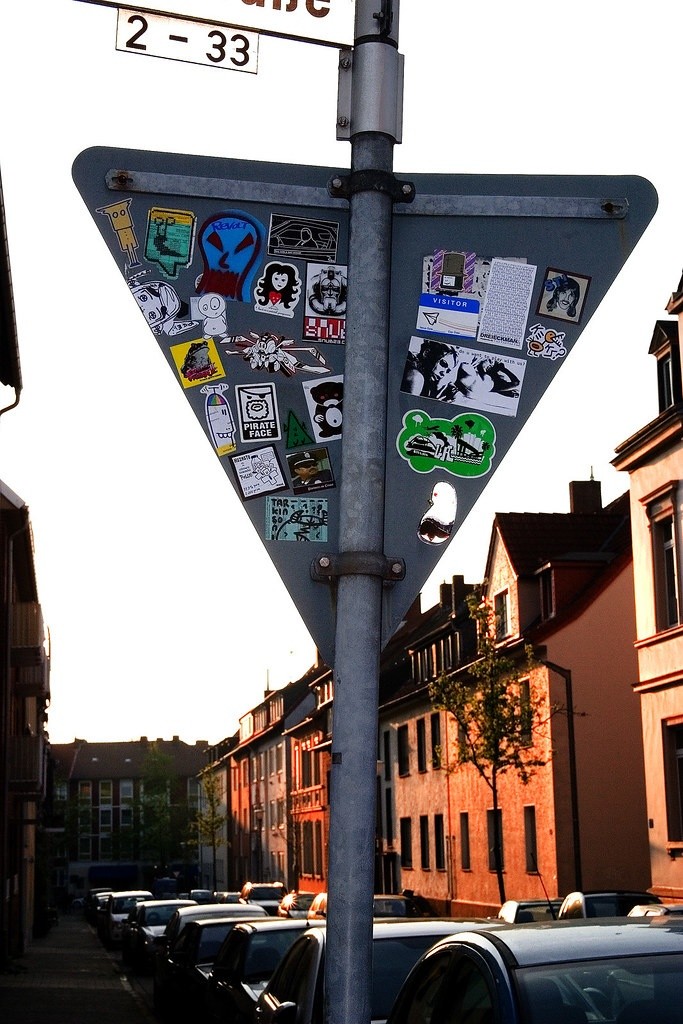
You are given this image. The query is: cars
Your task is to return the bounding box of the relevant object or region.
[385,915,682,1024]
[627,904,683,915]
[277,892,317,918]
[205,920,327,1024]
[154,903,270,969]
[190,889,212,905]
[252,917,503,1024]
[83,888,113,927]
[121,899,200,961]
[373,895,425,917]
[498,899,565,923]
[101,889,156,944]
[558,891,664,920]
[164,915,288,1018]
[305,892,328,920]
[210,891,241,904]
[238,881,288,916]
